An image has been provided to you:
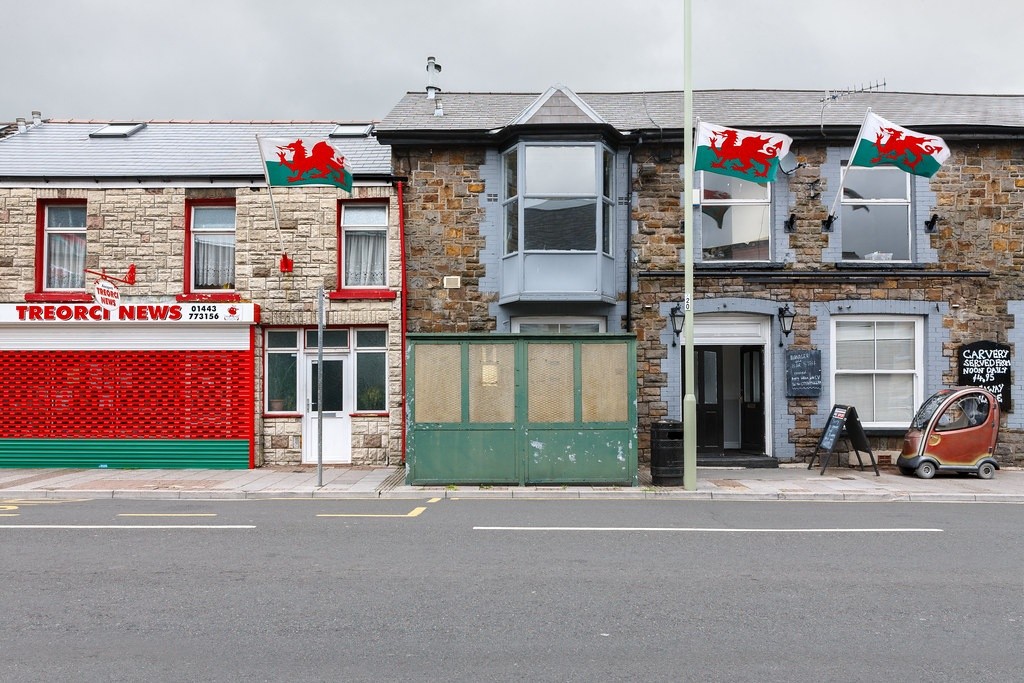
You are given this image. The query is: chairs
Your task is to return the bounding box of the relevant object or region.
[946,398,978,430]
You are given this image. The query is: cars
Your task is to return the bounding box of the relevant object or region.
[897,385,1002,480]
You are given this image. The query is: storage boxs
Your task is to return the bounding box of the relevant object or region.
[865,252,893,260]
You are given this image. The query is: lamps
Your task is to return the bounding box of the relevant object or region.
[777,304,797,348]
[669,301,686,348]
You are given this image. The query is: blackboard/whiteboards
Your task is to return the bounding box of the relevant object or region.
[818,405,848,451]
[785,349,821,397]
[957,339,1012,413]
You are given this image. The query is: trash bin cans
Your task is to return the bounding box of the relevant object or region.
[649,419,683,487]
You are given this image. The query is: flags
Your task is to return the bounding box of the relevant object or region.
[848,105,952,180]
[690,115,793,184]
[256,134,353,196]
[699,187,734,230]
[842,187,871,213]
